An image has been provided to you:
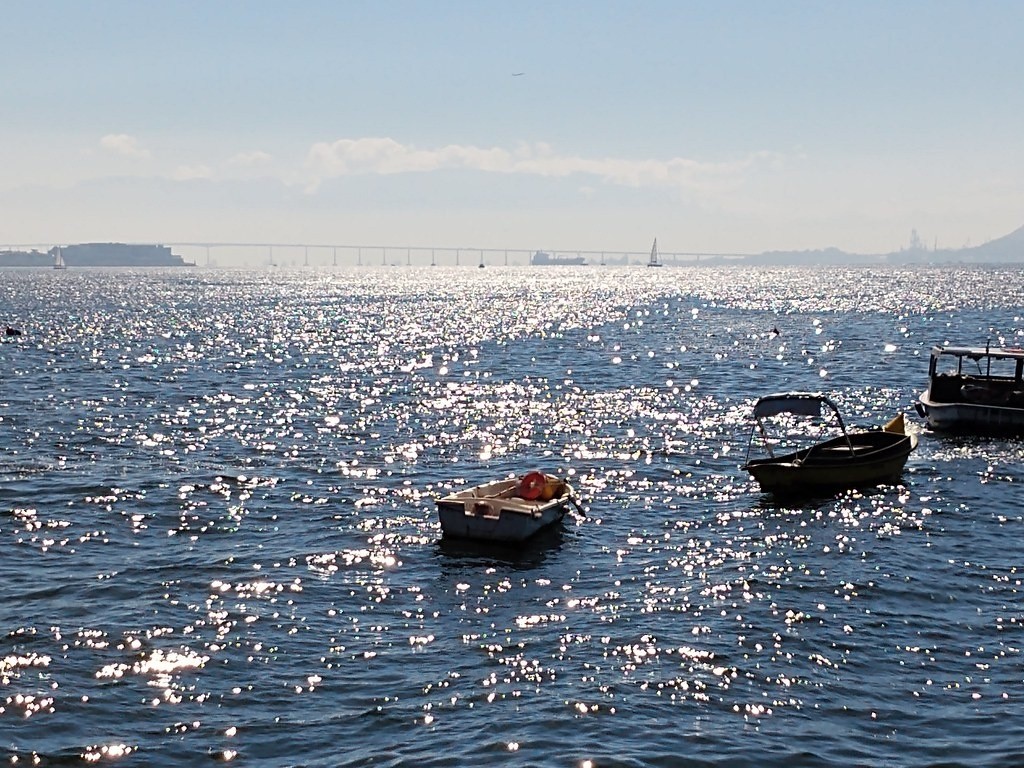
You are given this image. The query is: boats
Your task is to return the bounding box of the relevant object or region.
[531,249,589,265]
[434,469,576,543]
[740,392,919,495]
[915,336,1024,432]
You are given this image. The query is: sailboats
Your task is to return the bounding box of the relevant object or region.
[647,237,663,266]
